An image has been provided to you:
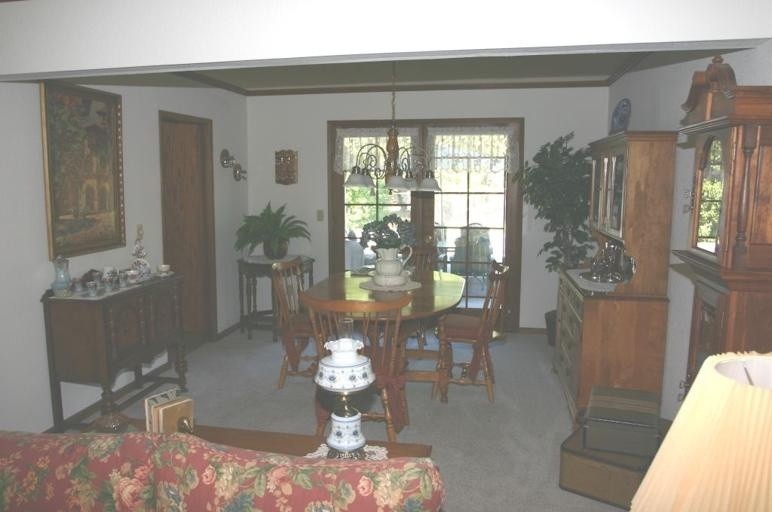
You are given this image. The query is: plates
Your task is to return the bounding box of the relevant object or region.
[612,98,631,132]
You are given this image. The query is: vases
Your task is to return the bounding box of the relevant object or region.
[368,243,416,289]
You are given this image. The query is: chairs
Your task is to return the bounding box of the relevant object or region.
[297,288,416,443]
[267,258,324,390]
[430,254,511,403]
[369,236,438,359]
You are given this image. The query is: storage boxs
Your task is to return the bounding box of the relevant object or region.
[577,386,666,457]
[558,418,674,509]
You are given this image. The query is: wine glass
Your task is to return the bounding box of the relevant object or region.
[592,241,634,282]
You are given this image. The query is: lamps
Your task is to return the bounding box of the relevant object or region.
[342,62,444,197]
[626,348,772,512]
[307,333,378,466]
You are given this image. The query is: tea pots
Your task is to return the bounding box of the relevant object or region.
[376,245,412,277]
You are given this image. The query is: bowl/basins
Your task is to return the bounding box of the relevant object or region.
[368,271,412,286]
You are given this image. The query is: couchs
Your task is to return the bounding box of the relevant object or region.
[2,423,448,510]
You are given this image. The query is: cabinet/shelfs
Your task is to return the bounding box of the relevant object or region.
[554,262,669,433]
[667,262,767,405]
[36,262,191,435]
[584,131,681,294]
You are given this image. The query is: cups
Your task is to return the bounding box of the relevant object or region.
[73,258,174,297]
[339,318,353,339]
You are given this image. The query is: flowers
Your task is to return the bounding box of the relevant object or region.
[358,212,417,251]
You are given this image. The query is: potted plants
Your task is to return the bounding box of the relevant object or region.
[513,128,593,345]
[232,200,314,261]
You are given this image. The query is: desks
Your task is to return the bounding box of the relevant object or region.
[106,416,432,464]
[290,267,469,403]
[232,253,314,344]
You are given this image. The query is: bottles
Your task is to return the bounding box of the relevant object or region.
[52,253,72,288]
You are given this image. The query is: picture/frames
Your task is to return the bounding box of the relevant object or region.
[38,79,129,262]
